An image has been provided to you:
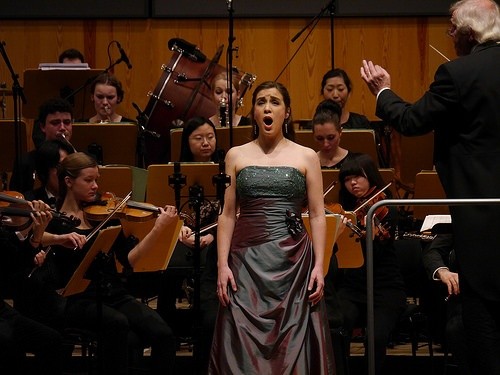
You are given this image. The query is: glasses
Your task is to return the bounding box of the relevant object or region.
[448,26,456,37]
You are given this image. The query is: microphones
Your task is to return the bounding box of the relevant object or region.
[115,41,132,69]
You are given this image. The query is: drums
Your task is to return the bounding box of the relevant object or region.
[141,45,255,161]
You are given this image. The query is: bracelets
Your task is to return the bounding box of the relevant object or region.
[31,238,40,243]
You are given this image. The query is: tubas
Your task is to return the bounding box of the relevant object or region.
[217,98,232,130]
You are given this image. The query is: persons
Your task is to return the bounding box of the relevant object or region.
[156,116,227,375]
[208,81,336,375]
[321,68,371,129]
[422,231,465,375]
[360,0,500,375]
[33,151,178,375]
[325,152,409,375]
[8,99,75,193]
[312,99,354,170]
[208,70,253,128]
[73,74,137,123]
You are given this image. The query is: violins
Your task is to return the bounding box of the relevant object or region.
[83,190,196,227]
[1,191,65,218]
[1,202,81,232]
[350,186,393,244]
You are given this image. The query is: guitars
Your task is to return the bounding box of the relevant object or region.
[394,225,439,244]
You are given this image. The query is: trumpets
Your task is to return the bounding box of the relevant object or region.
[56,132,79,155]
[97,105,114,125]
